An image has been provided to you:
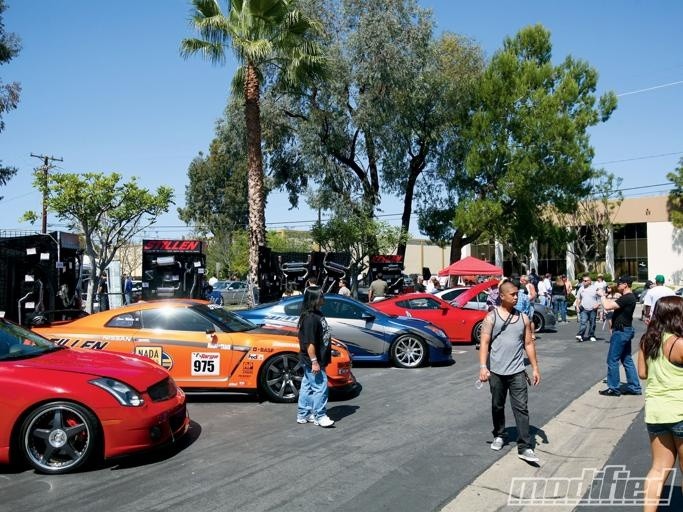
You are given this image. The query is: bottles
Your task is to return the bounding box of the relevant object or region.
[475,370,492,392]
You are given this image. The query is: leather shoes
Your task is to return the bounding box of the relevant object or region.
[598,388,621,397]
[621,389,641,396]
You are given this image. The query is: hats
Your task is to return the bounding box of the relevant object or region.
[613,275,634,284]
[654,274,667,284]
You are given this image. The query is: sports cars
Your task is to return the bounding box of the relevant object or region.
[1,307,199,482]
[361,274,502,348]
[22,291,356,406]
[229,291,457,370]
[432,282,558,336]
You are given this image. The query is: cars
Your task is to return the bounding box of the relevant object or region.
[207,277,249,307]
[632,286,644,303]
[126,280,143,298]
[671,287,682,298]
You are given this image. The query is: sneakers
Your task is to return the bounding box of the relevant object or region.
[590,336,597,342]
[517,448,540,464]
[313,413,335,428]
[575,335,583,342]
[296,413,315,424]
[490,437,504,452]
